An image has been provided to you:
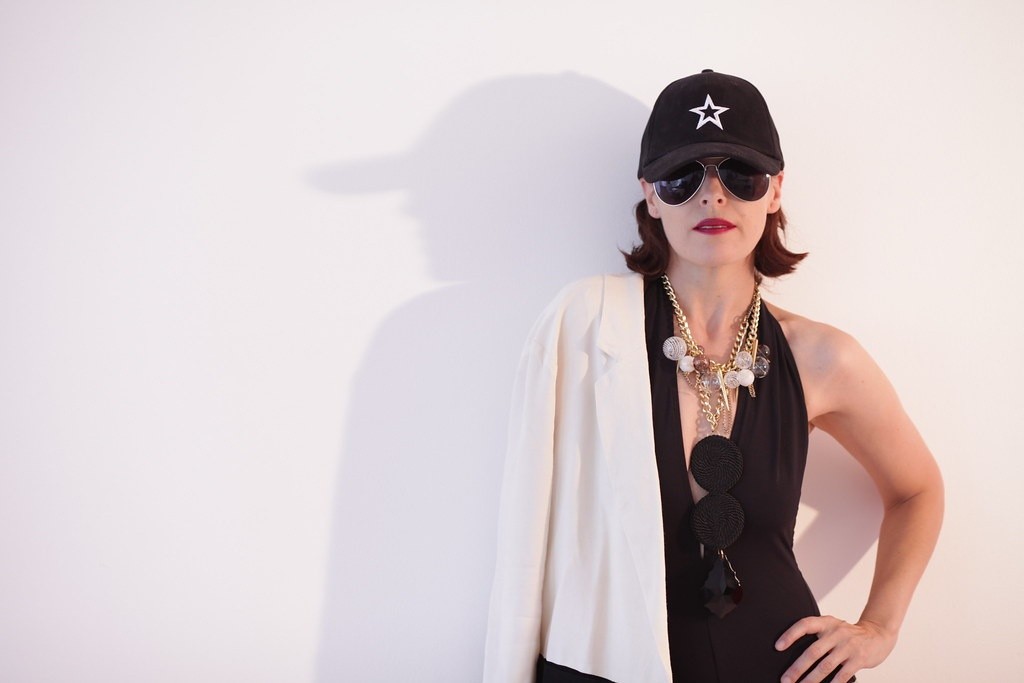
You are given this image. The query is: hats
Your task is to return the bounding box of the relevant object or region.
[638,69,785,183]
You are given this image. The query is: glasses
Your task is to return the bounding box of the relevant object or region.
[652,156,772,207]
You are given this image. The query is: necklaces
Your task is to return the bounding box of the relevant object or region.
[659,272,761,622]
[479,70,944,681]
